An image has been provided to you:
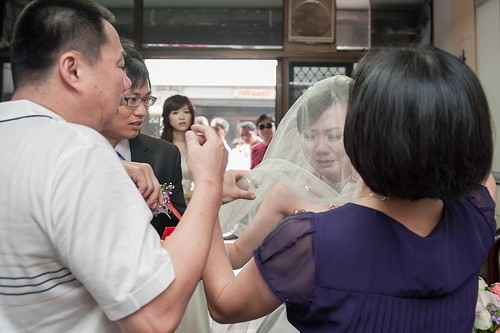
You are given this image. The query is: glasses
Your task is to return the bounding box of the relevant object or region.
[122,94,157,108]
[259,122,275,130]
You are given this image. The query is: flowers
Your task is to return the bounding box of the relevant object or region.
[152,181,175,221]
[472,276,500,333]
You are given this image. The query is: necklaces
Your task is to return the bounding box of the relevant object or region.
[312,171,359,193]
[358,194,388,201]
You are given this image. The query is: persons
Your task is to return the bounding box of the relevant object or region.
[0,0,228,333]
[203,43,500,333]
[100,39,357,333]
